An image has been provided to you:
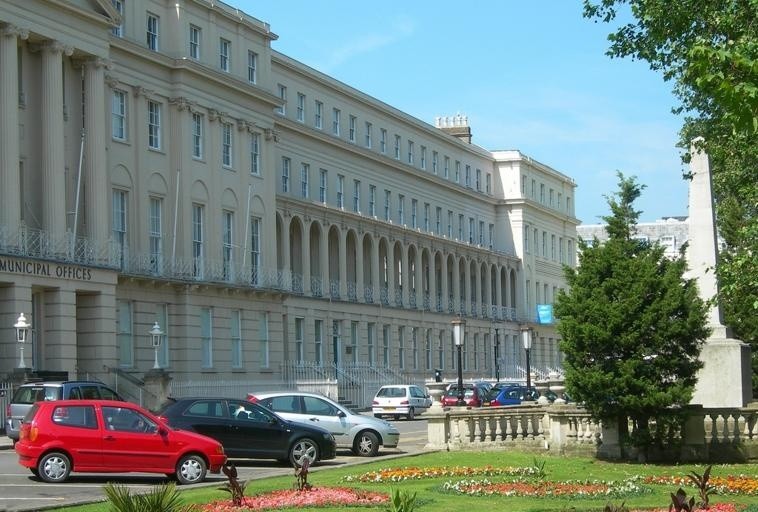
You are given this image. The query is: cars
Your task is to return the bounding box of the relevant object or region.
[441,382,541,412]
[372,384,432,421]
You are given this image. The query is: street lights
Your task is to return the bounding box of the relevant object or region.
[149,321,164,369]
[450,319,466,406]
[520,324,534,401]
[14,312,31,368]
